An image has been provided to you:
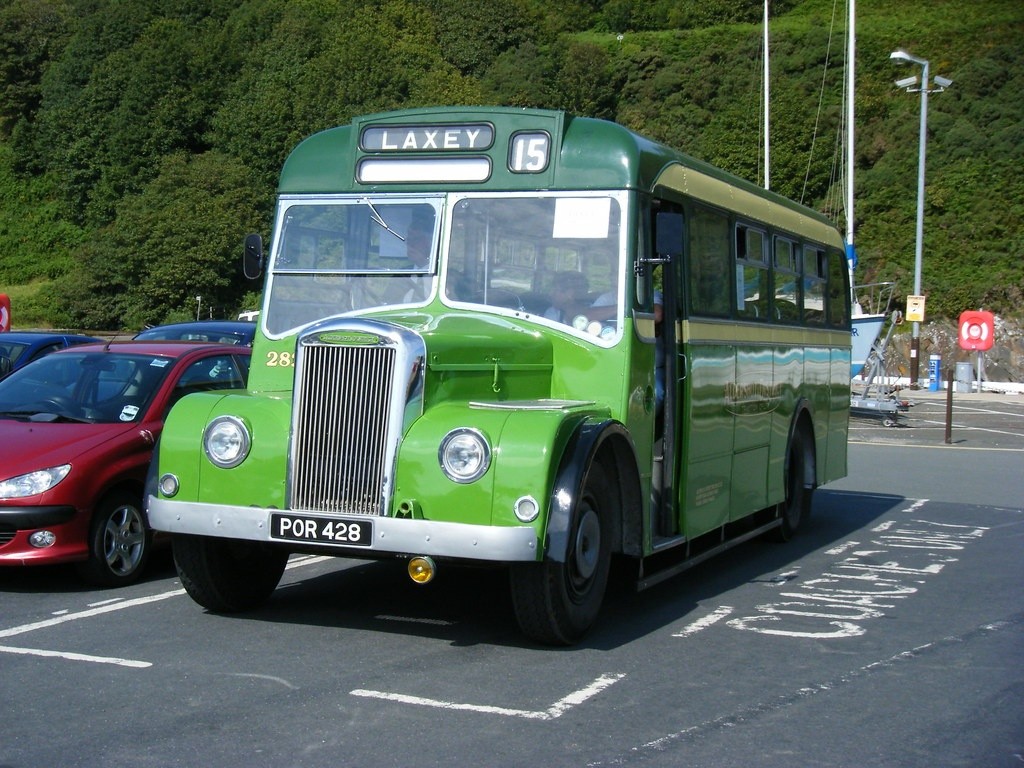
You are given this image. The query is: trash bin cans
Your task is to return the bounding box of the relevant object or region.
[955,361,973,393]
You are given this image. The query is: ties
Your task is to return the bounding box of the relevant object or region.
[411,274,424,303]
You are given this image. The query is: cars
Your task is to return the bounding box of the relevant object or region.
[0,343,251,589]
[0,333,108,385]
[129,322,255,350]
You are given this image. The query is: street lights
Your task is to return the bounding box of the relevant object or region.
[889,51,930,388]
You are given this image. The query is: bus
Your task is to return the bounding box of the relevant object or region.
[144,107,855,644]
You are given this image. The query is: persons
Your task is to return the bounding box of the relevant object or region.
[377,223,449,306]
[584,289,663,322]
[544,269,589,327]
